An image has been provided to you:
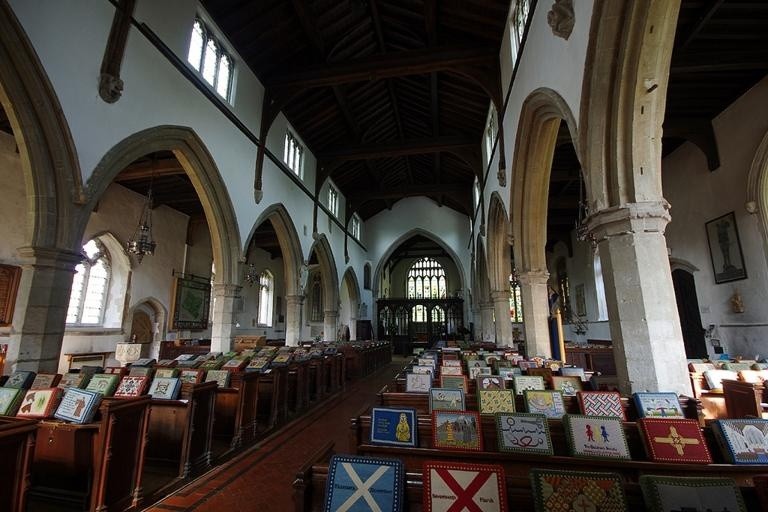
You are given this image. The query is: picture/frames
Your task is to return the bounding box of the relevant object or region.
[704,209,748,284]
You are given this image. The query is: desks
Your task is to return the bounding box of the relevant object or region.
[1,338,397,512]
[289,341,768,511]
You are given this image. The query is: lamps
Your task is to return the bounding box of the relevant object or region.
[126,152,160,265]
[244,263,260,288]
